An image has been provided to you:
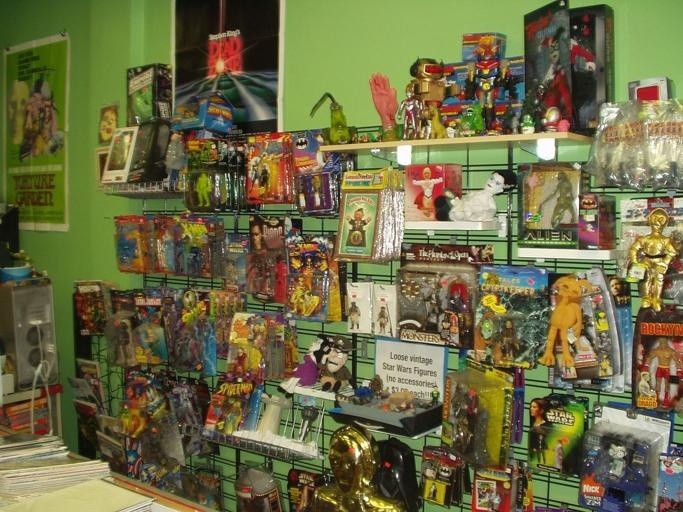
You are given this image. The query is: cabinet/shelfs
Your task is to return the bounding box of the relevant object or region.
[0,383,64,441]
[88,133,683,512]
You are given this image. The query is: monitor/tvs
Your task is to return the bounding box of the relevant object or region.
[0,204,20,268]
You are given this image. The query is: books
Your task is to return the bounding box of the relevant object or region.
[1,355,50,436]
[2,426,158,512]
[66,280,129,476]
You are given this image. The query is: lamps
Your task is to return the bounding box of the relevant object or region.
[396,145,412,166]
[534,138,556,161]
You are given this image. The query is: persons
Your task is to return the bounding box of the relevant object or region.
[111,51,678,512]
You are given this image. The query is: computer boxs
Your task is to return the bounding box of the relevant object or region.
[0,275,59,389]
